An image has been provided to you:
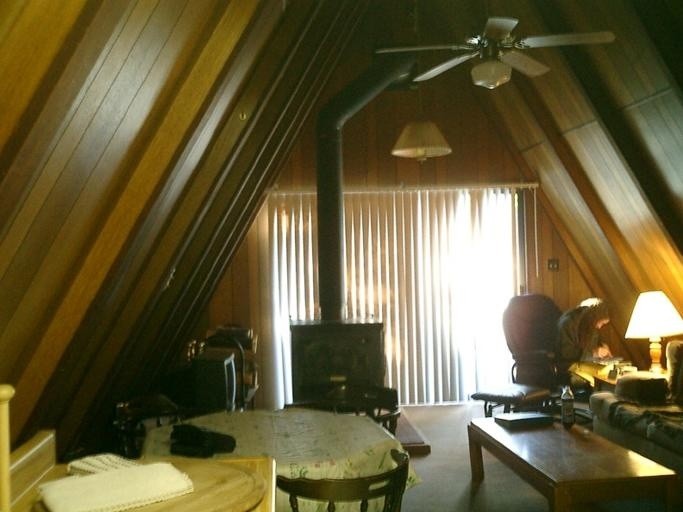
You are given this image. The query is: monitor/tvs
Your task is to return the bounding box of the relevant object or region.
[193,353,237,412]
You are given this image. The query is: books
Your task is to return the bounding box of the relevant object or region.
[592,355,623,366]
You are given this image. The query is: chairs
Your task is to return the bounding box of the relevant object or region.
[502,294,586,408]
[112,383,408,511]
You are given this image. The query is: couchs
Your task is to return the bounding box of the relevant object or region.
[588,340,683,476]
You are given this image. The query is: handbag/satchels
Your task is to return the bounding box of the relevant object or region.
[106,404,146,458]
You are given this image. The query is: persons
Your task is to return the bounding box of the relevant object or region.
[549,294,615,400]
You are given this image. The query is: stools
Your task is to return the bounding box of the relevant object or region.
[471,382,550,417]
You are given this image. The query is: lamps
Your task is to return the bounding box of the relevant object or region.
[470,42,512,90]
[389,1,453,164]
[624,290,683,374]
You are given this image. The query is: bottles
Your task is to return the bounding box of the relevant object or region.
[560,385,576,431]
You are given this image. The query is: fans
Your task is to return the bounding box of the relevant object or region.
[374,0,616,83]
